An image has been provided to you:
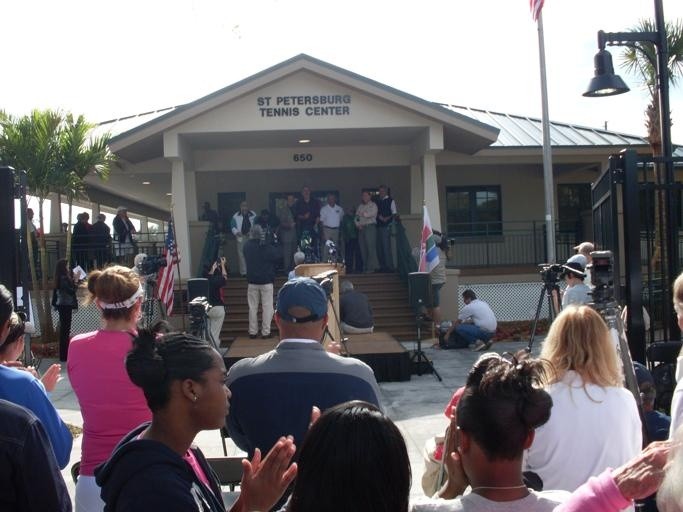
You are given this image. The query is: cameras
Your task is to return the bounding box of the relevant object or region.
[216,256,222,266]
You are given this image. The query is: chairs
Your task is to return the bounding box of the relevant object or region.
[202,456,251,511]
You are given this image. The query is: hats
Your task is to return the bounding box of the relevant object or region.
[560,262,583,278]
[276,277,327,323]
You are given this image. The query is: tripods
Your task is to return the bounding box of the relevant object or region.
[318,294,351,357]
[410,308,442,381]
[586,300,651,447]
[528,283,563,353]
[190,313,219,351]
[142,275,166,329]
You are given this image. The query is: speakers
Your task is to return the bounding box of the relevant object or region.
[409,272,431,308]
[186,278,210,312]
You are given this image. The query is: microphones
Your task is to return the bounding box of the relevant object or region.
[325,240,345,267]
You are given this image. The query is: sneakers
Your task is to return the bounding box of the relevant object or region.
[471,339,493,351]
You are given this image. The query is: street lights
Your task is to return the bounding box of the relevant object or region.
[583,0,683,348]
